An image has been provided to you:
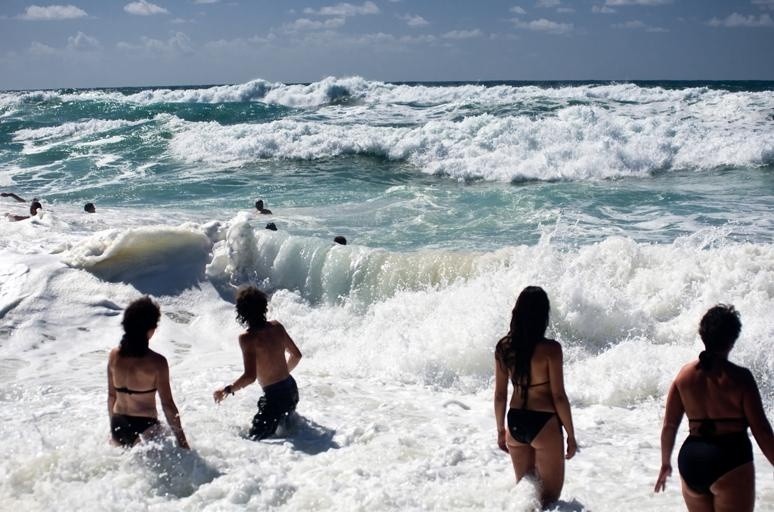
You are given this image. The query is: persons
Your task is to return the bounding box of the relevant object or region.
[653,301,774,511]
[82,202,96,212]
[213,286,305,443]
[492,285,578,511]
[1,191,40,204]
[265,222,278,230]
[334,236,346,245]
[2,200,41,223]
[255,199,271,213]
[105,295,192,450]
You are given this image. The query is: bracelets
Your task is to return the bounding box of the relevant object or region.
[222,386,232,394]
